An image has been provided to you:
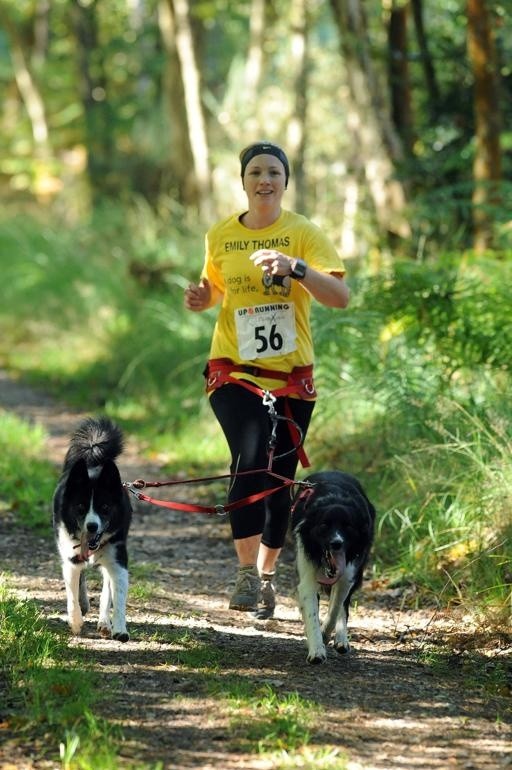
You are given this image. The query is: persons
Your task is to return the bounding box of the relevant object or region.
[182,144,348,619]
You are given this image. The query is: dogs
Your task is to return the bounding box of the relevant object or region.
[289,471,376,667]
[51,414,133,643]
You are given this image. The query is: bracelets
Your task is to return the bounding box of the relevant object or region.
[291,256,307,281]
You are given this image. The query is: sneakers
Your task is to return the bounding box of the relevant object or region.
[230,563,264,611]
[254,570,280,620]
